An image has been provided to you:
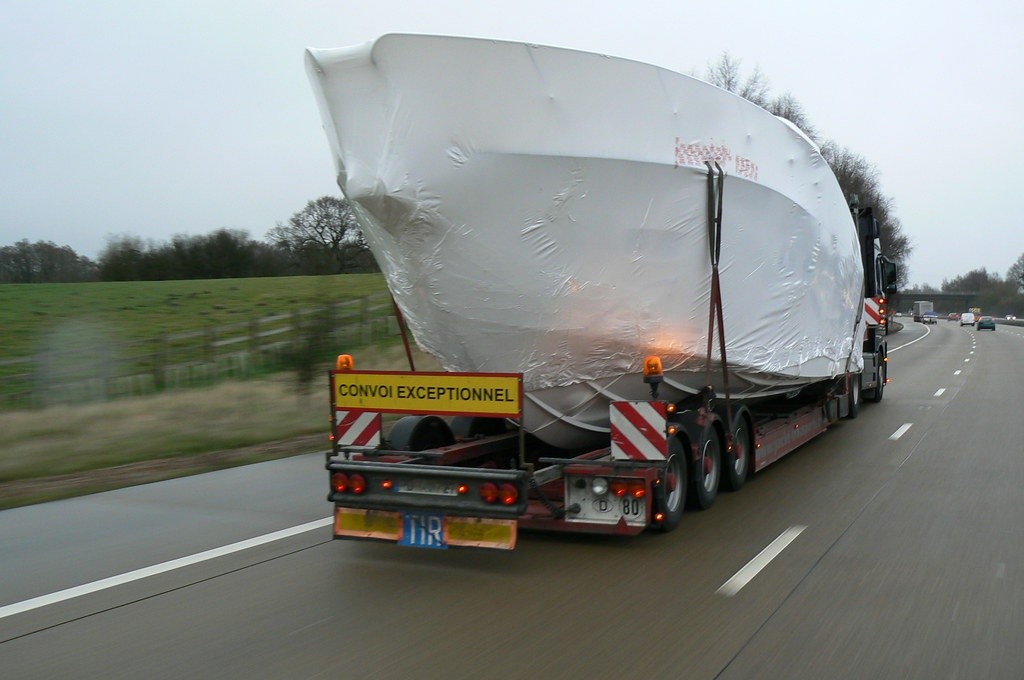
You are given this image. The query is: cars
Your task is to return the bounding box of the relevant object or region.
[895,313,901,317]
[947,313,958,321]
[976,316,995,332]
[1005,314,1016,320]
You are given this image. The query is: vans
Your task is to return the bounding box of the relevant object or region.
[960,313,975,326]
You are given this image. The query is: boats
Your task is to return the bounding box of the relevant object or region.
[300,32,867,457]
[923,312,937,322]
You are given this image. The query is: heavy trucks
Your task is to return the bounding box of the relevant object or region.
[911,300,933,322]
[327,212,899,552]
